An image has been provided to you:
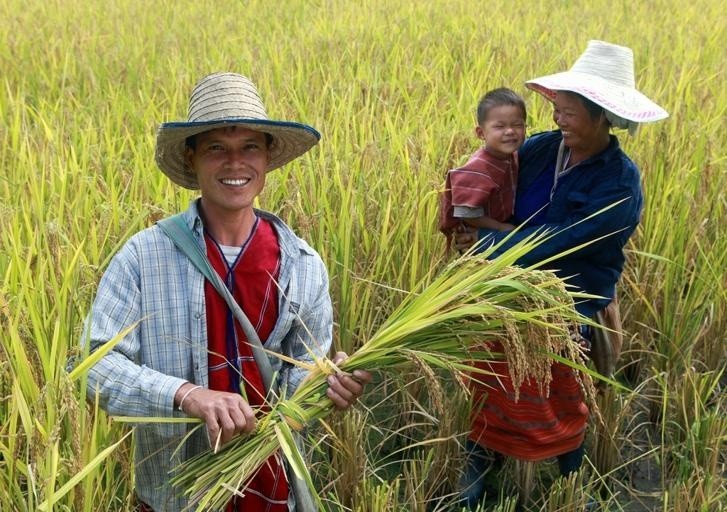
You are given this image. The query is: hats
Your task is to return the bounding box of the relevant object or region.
[527,38,671,126]
[155,72,321,191]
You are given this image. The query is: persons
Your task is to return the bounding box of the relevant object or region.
[452,40,670,512]
[441,88,529,245]
[66,127,372,512]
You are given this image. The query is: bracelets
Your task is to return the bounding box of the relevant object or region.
[178,385,203,411]
